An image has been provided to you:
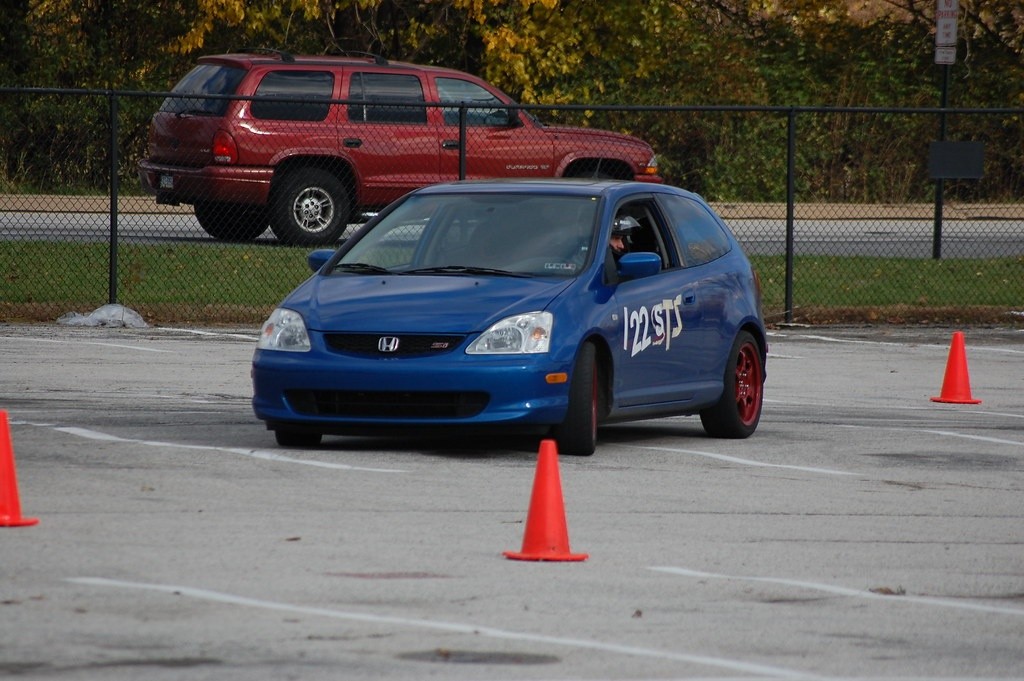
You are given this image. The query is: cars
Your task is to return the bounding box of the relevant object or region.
[251,176,770,458]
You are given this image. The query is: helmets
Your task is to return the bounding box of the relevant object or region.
[577,201,640,264]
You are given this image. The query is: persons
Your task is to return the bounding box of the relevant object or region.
[606,212,640,255]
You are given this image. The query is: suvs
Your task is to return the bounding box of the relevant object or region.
[133,48,666,249]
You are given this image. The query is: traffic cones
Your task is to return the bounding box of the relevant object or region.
[503,436,591,562]
[0,408,42,528]
[929,329,983,404]
